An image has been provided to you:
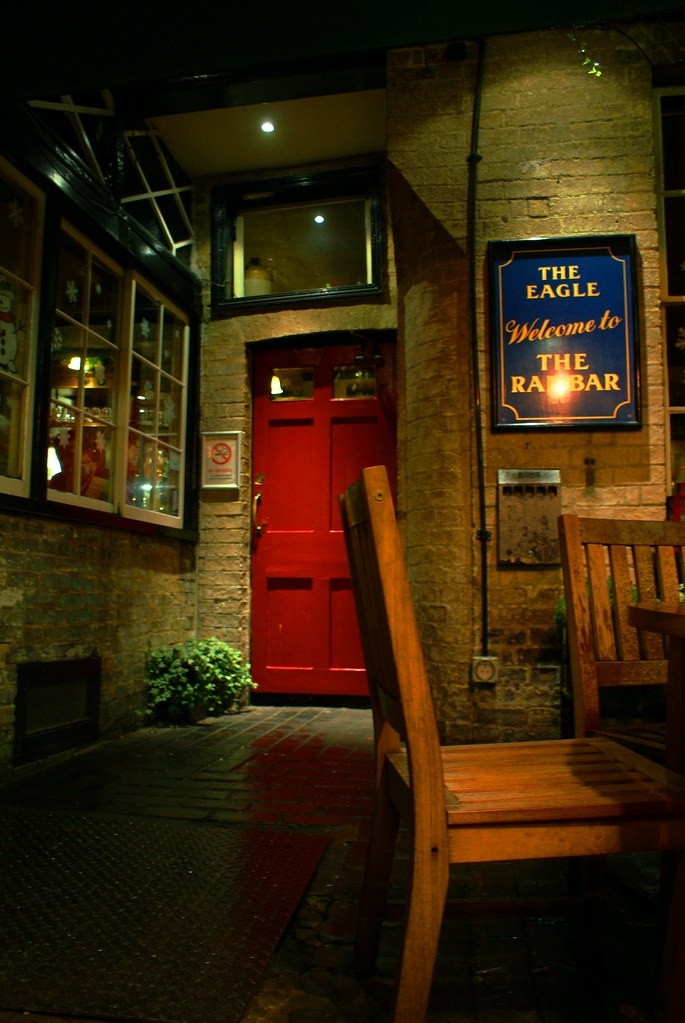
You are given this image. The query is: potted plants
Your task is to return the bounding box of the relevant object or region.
[141,636,259,726]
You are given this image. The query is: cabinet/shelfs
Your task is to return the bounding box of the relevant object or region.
[48,337,177,517]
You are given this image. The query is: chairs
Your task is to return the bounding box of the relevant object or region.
[337,465,685,1023]
[556,515,685,770]
